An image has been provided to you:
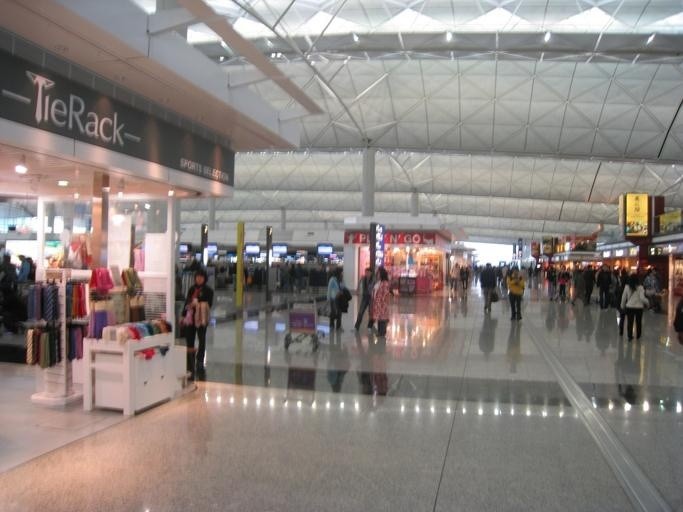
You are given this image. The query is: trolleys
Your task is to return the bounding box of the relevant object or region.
[284,294,325,354]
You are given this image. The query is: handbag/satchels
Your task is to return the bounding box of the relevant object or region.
[490,293,499,303]
[317,306,332,318]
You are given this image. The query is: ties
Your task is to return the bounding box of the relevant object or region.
[20,282,61,369]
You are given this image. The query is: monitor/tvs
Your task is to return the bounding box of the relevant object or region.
[180,244,190,253]
[317,243,333,255]
[245,245,260,256]
[271,246,288,256]
[208,244,217,255]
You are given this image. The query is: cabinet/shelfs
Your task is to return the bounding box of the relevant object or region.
[27,280,88,407]
[82,335,188,417]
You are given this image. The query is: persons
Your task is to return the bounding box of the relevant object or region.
[26,258,36,282]
[183,271,214,380]
[493,265,509,298]
[232,262,328,291]
[350,268,376,333]
[17,255,30,281]
[547,260,661,341]
[506,267,525,320]
[0,253,16,282]
[370,266,390,339]
[527,262,543,290]
[449,263,485,292]
[480,262,496,312]
[327,267,345,332]
